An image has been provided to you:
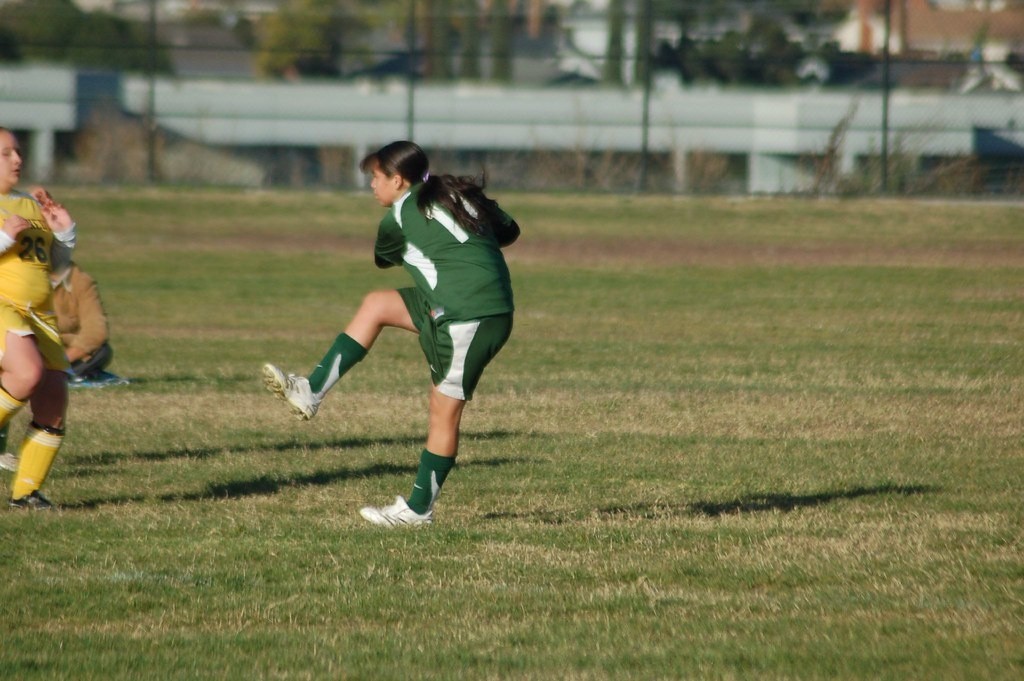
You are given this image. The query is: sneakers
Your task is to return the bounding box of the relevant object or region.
[262,364,321,421]
[10,490,53,511]
[359,495,433,529]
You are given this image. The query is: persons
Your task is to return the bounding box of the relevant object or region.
[0,127,114,513]
[262,141,521,528]
